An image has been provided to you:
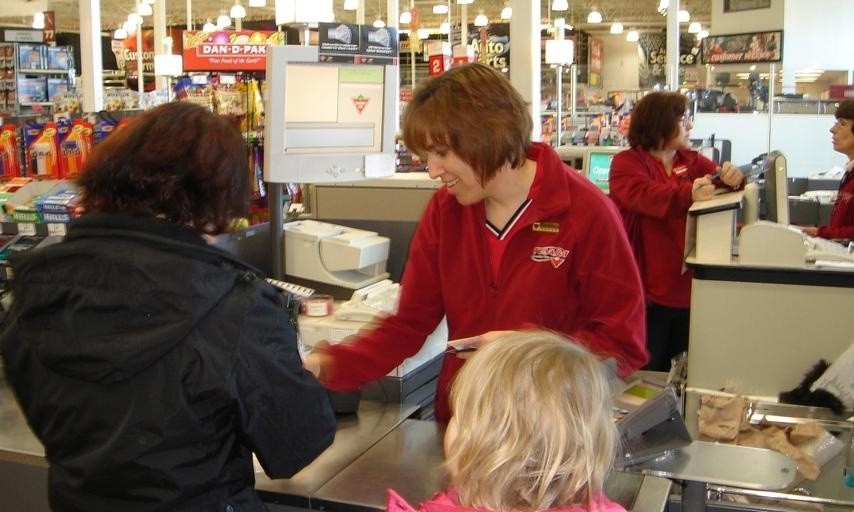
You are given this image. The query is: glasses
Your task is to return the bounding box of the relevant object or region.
[835,121,851,127]
[679,115,693,127]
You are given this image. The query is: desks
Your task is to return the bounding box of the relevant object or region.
[684,179,854,417]
[0,354,677,512]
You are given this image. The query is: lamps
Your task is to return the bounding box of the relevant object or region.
[609,17,624,35]
[678,7,691,23]
[626,24,640,42]
[399,11,410,24]
[688,8,701,33]
[217,9,232,28]
[474,12,489,26]
[552,1,569,11]
[587,3,602,23]
[230,1,247,18]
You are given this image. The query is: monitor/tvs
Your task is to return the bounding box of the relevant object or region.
[765,150,790,225]
[263,45,400,187]
[584,148,622,194]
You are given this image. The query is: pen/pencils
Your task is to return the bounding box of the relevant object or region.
[693,174,719,190]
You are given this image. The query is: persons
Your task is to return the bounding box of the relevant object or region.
[801,98,854,243]
[301,60,652,422]
[0,100,338,511]
[607,91,746,372]
[386,332,627,511]
